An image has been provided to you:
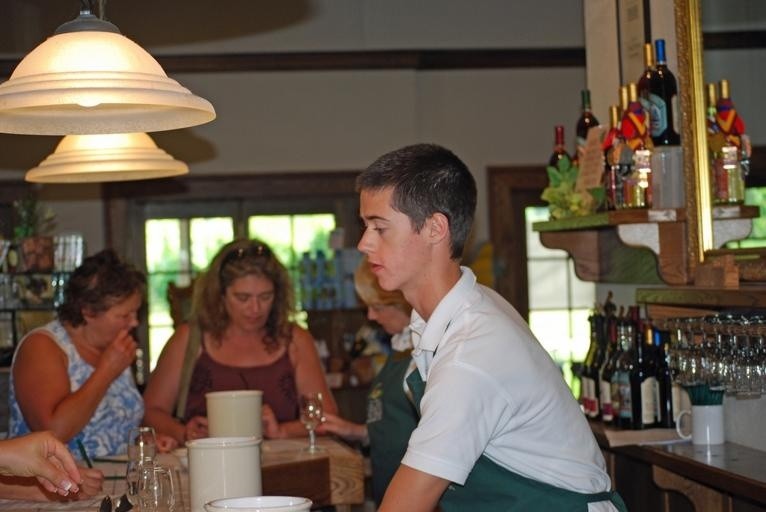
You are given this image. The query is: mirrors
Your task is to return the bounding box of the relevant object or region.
[674,0,766,285]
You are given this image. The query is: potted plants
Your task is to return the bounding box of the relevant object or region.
[12,196,59,271]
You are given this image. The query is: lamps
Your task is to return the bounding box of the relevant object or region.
[24,0,189,183]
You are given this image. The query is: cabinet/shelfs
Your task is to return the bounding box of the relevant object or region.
[589,421,680,491]
[303,307,370,424]
[613,443,766,512]
[0,271,72,369]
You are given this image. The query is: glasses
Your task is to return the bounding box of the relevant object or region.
[230,243,270,260]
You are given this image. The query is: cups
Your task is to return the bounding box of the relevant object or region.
[651,144,686,211]
[138,465,176,511]
[185,435,265,512]
[202,494,314,512]
[664,312,766,399]
[298,391,326,456]
[674,403,726,446]
[204,389,264,439]
[126,426,159,464]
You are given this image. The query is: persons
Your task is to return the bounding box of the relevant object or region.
[7,245,177,463]
[142,240,337,446]
[0,431,105,502]
[359,144,628,512]
[313,255,426,512]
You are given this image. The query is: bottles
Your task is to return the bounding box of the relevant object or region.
[582,305,673,430]
[620,86,629,121]
[638,45,656,111]
[710,80,751,176]
[648,40,680,146]
[546,126,573,183]
[706,82,718,131]
[577,88,599,165]
[603,105,620,153]
[621,84,652,150]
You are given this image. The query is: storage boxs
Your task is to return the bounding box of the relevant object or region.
[0,0,216,136]
